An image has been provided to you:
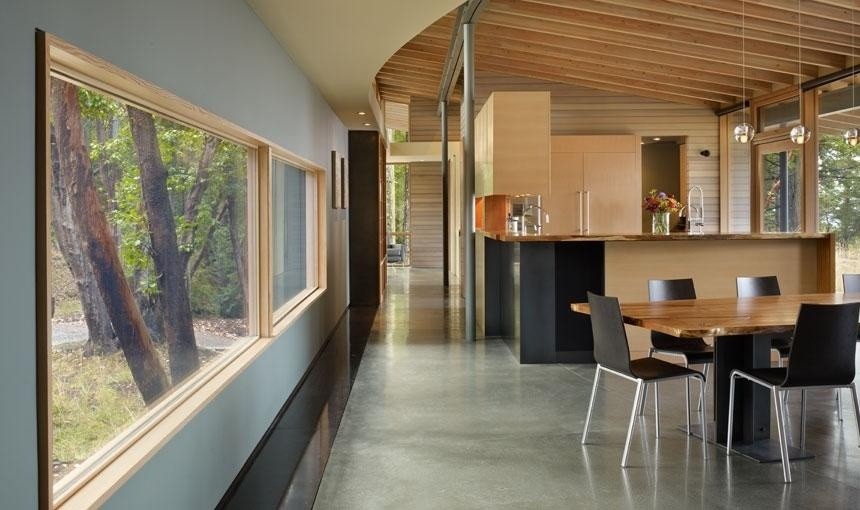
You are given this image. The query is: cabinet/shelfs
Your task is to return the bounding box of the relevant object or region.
[550,134,643,235]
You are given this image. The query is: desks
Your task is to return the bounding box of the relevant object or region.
[570,292,860,464]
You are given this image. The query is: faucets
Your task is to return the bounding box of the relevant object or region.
[524,221,537,235]
[520,205,550,235]
[676,204,701,217]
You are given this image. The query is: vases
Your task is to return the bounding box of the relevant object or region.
[651,212,670,235]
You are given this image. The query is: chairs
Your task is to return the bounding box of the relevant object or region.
[388,242,407,268]
[726,302,859,483]
[638,277,715,413]
[841,273,860,294]
[580,291,709,470]
[735,276,793,368]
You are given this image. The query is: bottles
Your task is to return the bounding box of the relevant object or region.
[511,217,519,232]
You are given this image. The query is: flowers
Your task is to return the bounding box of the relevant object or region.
[644,188,685,233]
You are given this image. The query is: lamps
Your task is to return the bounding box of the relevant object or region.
[732,1,859,149]
